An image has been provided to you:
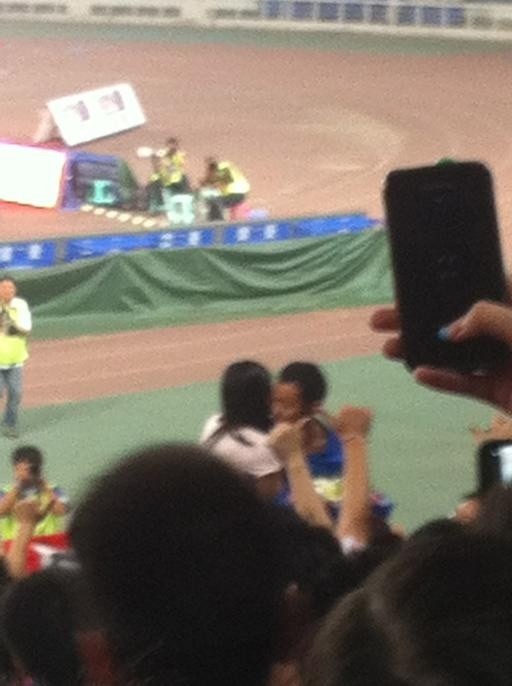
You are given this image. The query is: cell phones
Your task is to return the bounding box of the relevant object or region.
[382,160,508,373]
[478,438,512,493]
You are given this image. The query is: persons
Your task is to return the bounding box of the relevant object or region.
[143,140,250,223]
[1,276,32,439]
[1,446,74,552]
[272,360,395,525]
[0,408,511,686]
[199,360,285,498]
[368,276,512,419]
